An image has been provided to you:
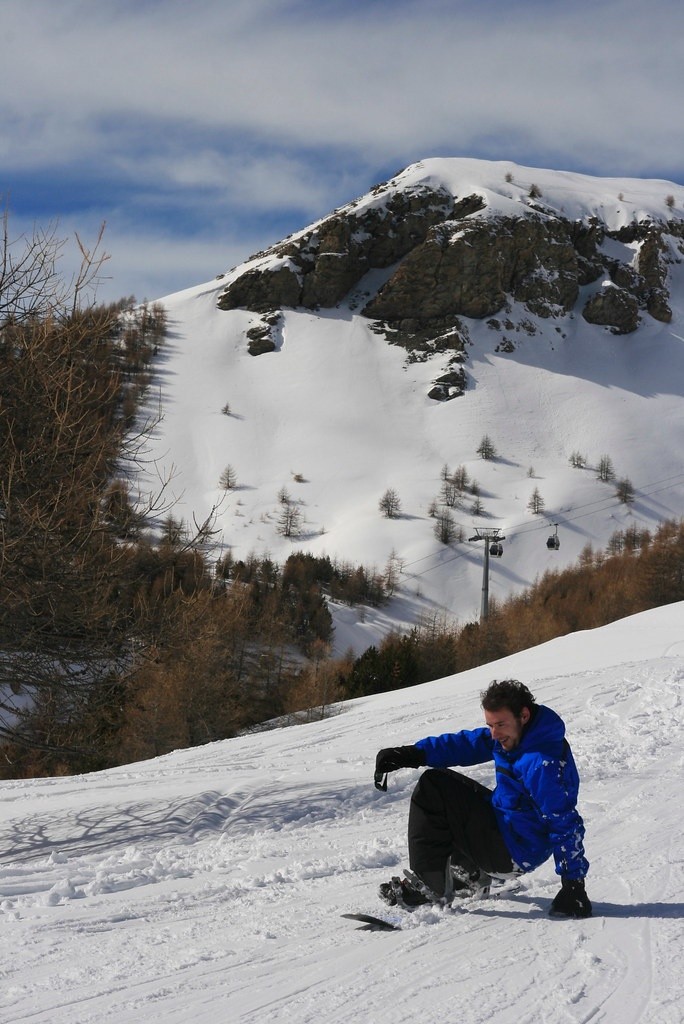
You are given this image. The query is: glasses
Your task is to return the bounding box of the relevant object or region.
[374,761,400,792]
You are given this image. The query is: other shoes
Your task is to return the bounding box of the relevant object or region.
[452,876,469,889]
[379,878,432,907]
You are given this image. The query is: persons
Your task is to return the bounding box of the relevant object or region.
[376,678,594,918]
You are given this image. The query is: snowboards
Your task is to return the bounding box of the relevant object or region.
[339,881,522,930]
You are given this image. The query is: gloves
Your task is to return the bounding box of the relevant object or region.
[375,745,422,773]
[549,876,592,919]
